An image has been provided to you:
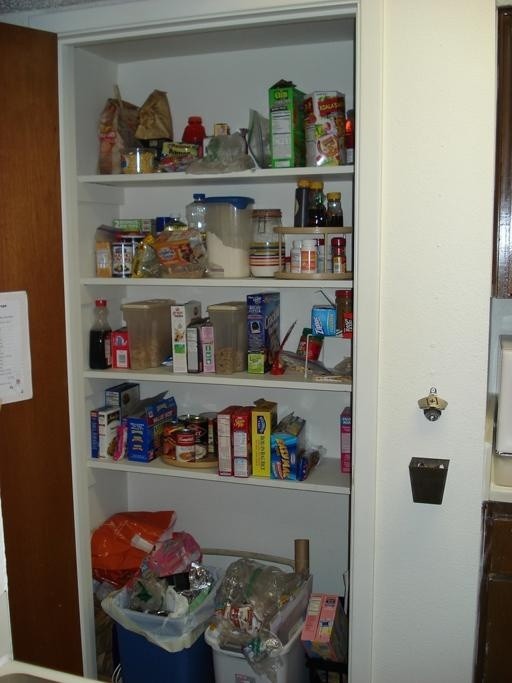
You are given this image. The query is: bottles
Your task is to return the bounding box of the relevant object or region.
[163,213,187,233]
[249,208,286,279]
[181,115,207,159]
[293,179,343,228]
[185,193,206,250]
[88,298,113,369]
[290,237,346,274]
[205,196,255,278]
[345,109,355,165]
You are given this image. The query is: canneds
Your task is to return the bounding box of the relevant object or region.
[163,412,218,462]
[296,328,324,361]
[111,235,144,277]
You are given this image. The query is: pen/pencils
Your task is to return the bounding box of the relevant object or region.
[272,319,298,366]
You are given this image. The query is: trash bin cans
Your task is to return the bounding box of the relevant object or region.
[102,566,308,683]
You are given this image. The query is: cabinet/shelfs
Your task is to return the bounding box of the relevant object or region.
[57,0,381,683]
